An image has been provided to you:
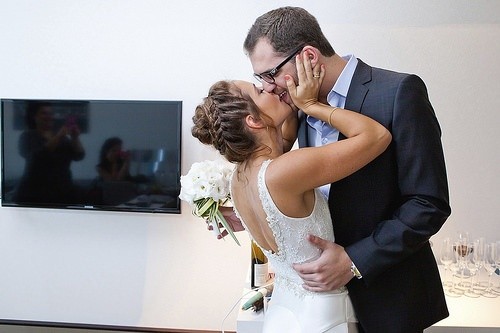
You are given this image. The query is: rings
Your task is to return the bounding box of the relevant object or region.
[313,75,319,76]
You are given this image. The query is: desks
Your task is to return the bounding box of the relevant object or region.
[421,263,500,333]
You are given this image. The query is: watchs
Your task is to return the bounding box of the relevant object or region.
[350,263,363,279]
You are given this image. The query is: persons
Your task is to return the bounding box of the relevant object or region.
[191,51,393,333]
[15,106,132,205]
[203,7,452,333]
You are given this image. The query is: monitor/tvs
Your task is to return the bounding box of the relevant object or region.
[0,98,182,215]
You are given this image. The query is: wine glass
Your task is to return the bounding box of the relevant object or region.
[438,233,500,297]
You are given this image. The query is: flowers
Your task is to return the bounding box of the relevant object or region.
[178,160,240,247]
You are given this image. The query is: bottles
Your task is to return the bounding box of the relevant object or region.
[251,239,268,287]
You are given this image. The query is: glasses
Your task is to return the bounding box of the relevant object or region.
[253,43,304,84]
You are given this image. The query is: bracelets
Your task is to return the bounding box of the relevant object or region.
[328,107,342,127]
